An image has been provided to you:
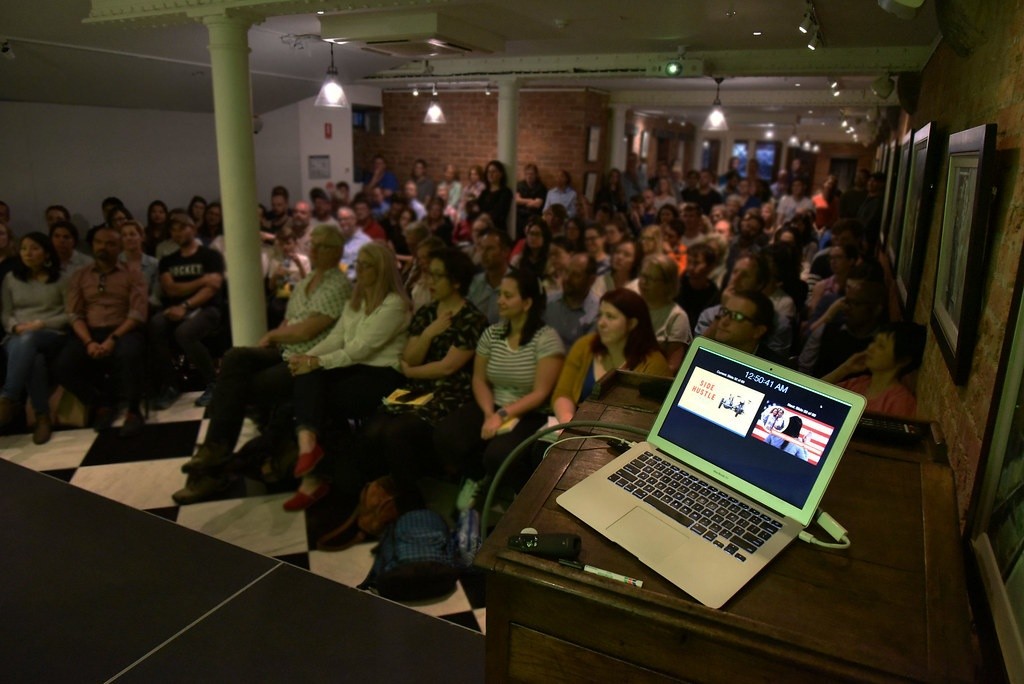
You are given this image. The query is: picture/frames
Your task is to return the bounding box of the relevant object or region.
[879,122,997,388]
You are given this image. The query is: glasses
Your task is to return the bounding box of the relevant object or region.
[97,274,107,293]
[717,306,758,326]
[352,260,376,272]
[582,234,600,243]
[428,269,447,282]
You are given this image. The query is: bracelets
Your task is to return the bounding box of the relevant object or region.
[497,408,509,422]
[307,357,314,372]
[85,339,93,346]
[109,333,119,342]
[182,300,191,309]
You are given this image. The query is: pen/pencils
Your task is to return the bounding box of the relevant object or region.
[557,558,644,590]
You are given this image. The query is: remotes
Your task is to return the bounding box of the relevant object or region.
[508,532,581,562]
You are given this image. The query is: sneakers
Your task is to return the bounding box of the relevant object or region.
[182,444,235,474]
[455,472,493,511]
[152,391,178,410]
[173,474,233,505]
[194,383,218,406]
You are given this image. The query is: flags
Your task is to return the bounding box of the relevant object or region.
[751,400,835,465]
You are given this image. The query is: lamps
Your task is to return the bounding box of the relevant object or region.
[314,43,349,108]
[808,31,820,50]
[799,12,813,33]
[701,77,729,131]
[424,85,446,124]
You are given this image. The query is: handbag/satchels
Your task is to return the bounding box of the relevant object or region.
[357,481,399,535]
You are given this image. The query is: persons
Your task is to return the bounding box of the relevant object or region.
[464,229,515,324]
[764,409,786,430]
[363,156,401,206]
[818,316,921,420]
[688,168,723,216]
[714,288,789,368]
[765,415,813,461]
[266,226,312,332]
[0,152,891,566]
[541,253,602,351]
[411,159,433,208]
[171,225,353,503]
[354,197,385,242]
[237,240,413,510]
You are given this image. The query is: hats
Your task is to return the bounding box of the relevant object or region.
[169,213,196,229]
[276,226,294,238]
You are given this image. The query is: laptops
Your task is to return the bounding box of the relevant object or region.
[556,336,868,610]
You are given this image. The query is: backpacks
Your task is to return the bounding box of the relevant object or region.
[357,509,467,602]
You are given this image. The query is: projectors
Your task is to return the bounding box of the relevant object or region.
[646,58,704,78]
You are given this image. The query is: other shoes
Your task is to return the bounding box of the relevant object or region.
[293,444,326,478]
[283,481,328,512]
[94,407,115,430]
[118,413,145,438]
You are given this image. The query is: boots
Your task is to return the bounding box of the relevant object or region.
[33,407,51,444]
[0,395,26,433]
